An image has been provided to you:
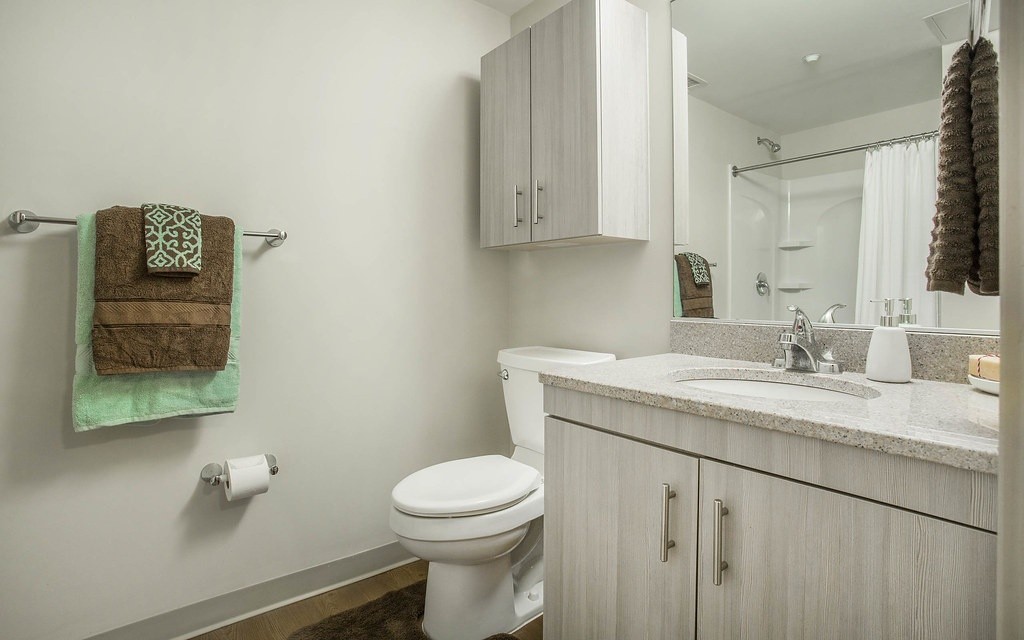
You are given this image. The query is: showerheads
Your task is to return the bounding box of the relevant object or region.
[757,136,781,152]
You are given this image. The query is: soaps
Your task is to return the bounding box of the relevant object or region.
[968,354,1001,381]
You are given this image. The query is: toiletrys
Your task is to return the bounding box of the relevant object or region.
[898,296,920,328]
[865,297,912,384]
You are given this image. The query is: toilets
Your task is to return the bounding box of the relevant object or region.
[388,345,618,640]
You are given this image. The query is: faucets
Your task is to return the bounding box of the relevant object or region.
[771,304,843,374]
[816,303,847,323]
[757,280,771,296]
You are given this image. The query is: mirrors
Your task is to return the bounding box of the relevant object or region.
[670,1,1002,339]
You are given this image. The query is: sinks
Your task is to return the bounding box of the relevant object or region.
[669,368,882,402]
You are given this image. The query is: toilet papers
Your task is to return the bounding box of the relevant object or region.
[223,454,271,502]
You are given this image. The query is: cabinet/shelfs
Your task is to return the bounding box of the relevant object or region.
[671,27,690,247]
[481,0,650,254]
[778,240,813,292]
[542,415,1000,640]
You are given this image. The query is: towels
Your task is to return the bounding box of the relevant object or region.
[69,202,243,433]
[672,252,714,318]
[924,36,1000,297]
